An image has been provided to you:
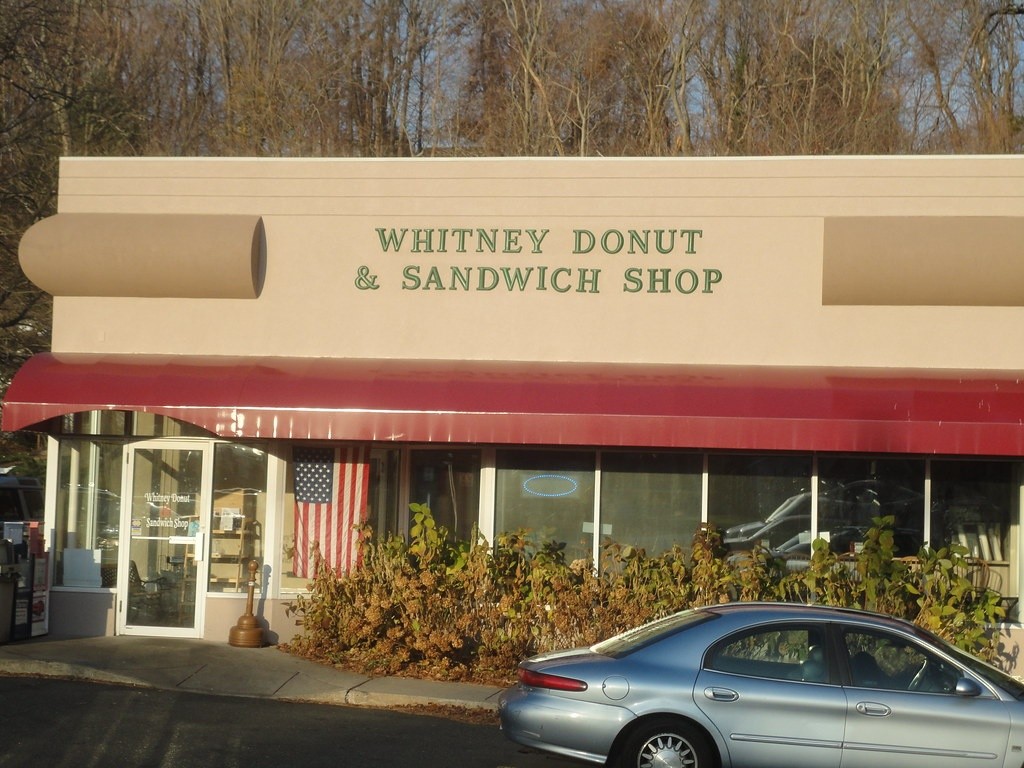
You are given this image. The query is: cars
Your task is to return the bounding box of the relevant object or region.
[0,475,120,566]
[723,479,952,582]
[495,600,1024,768]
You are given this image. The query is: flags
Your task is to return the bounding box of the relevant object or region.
[293,447,370,581]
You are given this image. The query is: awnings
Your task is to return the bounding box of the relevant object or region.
[2,353,1024,459]
